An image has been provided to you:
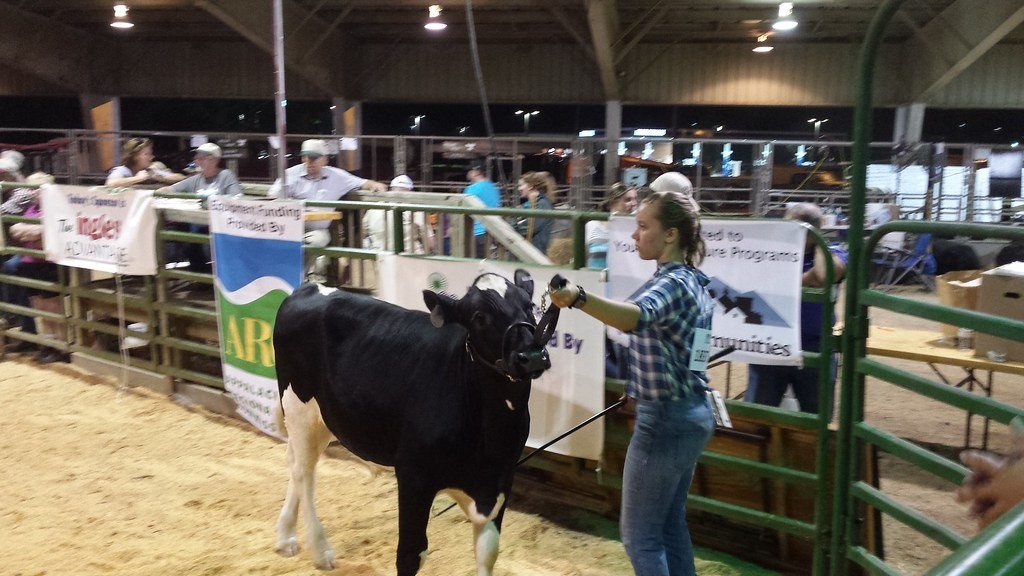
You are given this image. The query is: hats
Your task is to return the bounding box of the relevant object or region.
[462,159,485,173]
[650,170,701,212]
[188,143,223,161]
[390,173,415,190]
[295,139,330,158]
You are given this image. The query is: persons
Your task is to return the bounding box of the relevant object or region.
[268,139,388,247]
[587,183,638,269]
[752,201,848,423]
[509,172,555,263]
[0,150,71,363]
[632,173,702,214]
[157,142,242,234]
[954,450,1024,529]
[105,137,187,188]
[463,162,502,257]
[362,175,435,254]
[549,192,716,576]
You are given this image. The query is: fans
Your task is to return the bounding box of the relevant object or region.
[890,137,929,173]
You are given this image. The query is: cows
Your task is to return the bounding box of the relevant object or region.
[273,269,551,576]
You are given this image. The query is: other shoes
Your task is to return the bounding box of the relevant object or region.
[3,342,33,356]
[36,348,65,364]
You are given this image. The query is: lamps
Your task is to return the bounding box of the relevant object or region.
[109,2,134,29]
[424,4,448,30]
[772,3,798,30]
[753,34,775,53]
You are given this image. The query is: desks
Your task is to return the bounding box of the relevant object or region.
[833,320,1023,453]
[156,195,342,286]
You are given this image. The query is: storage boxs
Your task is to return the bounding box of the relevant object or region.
[935,267,1023,362]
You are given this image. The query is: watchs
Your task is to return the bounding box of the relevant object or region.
[568,285,586,309]
